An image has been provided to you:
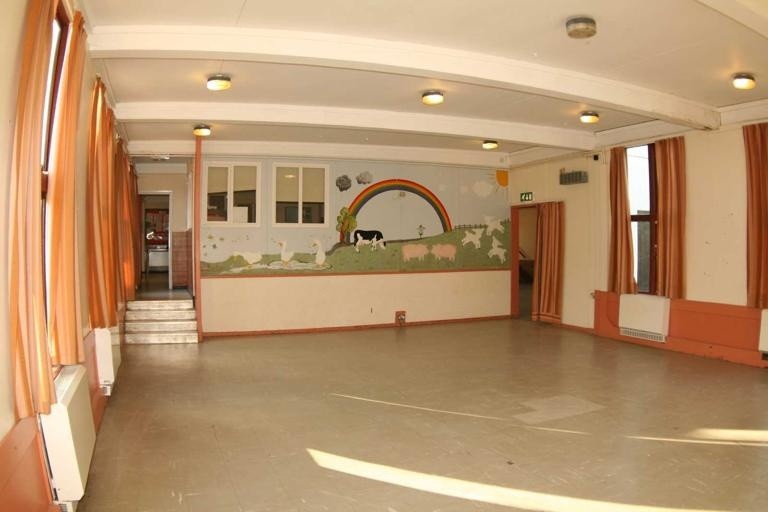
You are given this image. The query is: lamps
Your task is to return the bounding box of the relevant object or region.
[193,123,211,136]
[482,140,498,150]
[422,90,445,105]
[580,111,600,124]
[206,76,230,92]
[732,72,755,89]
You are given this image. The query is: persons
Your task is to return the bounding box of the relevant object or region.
[146,229,160,240]
